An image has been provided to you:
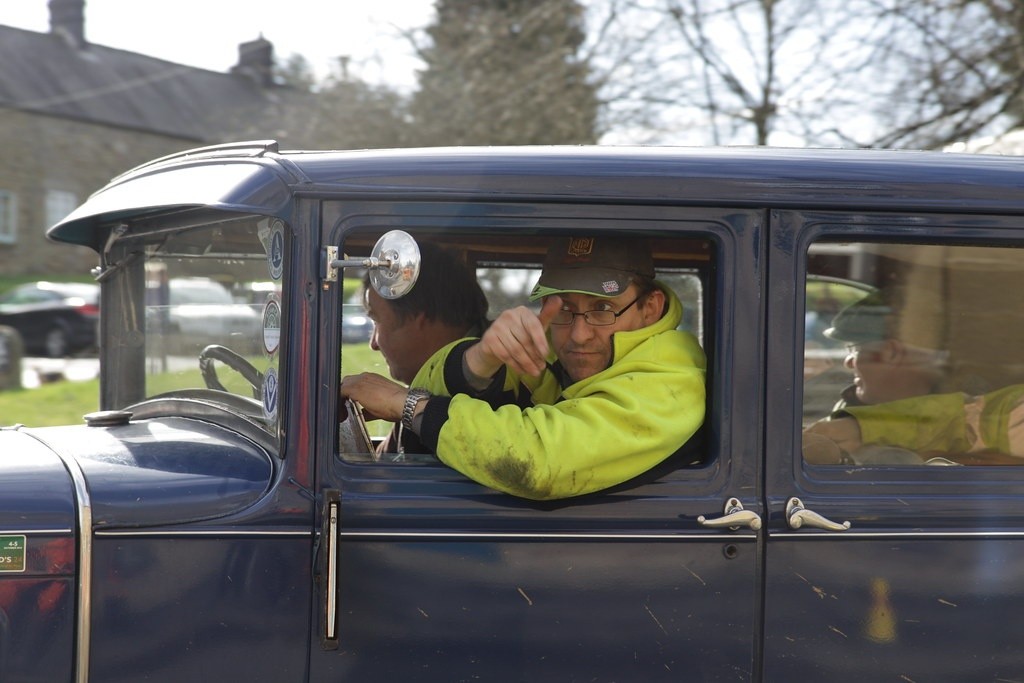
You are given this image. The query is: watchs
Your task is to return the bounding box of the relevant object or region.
[401,388,434,432]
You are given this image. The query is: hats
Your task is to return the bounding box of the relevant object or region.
[823,284,964,359]
[528,235,655,303]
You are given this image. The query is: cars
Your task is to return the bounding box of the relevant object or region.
[0,282,100,359]
[147,277,261,354]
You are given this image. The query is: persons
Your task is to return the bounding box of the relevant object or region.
[801,384,1024,469]
[823,287,1000,413]
[362,242,495,463]
[341,232,707,502]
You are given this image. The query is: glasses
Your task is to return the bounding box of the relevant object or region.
[846,336,892,358]
[539,290,648,325]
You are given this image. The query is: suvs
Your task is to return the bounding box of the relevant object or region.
[0,141,1024,683]
